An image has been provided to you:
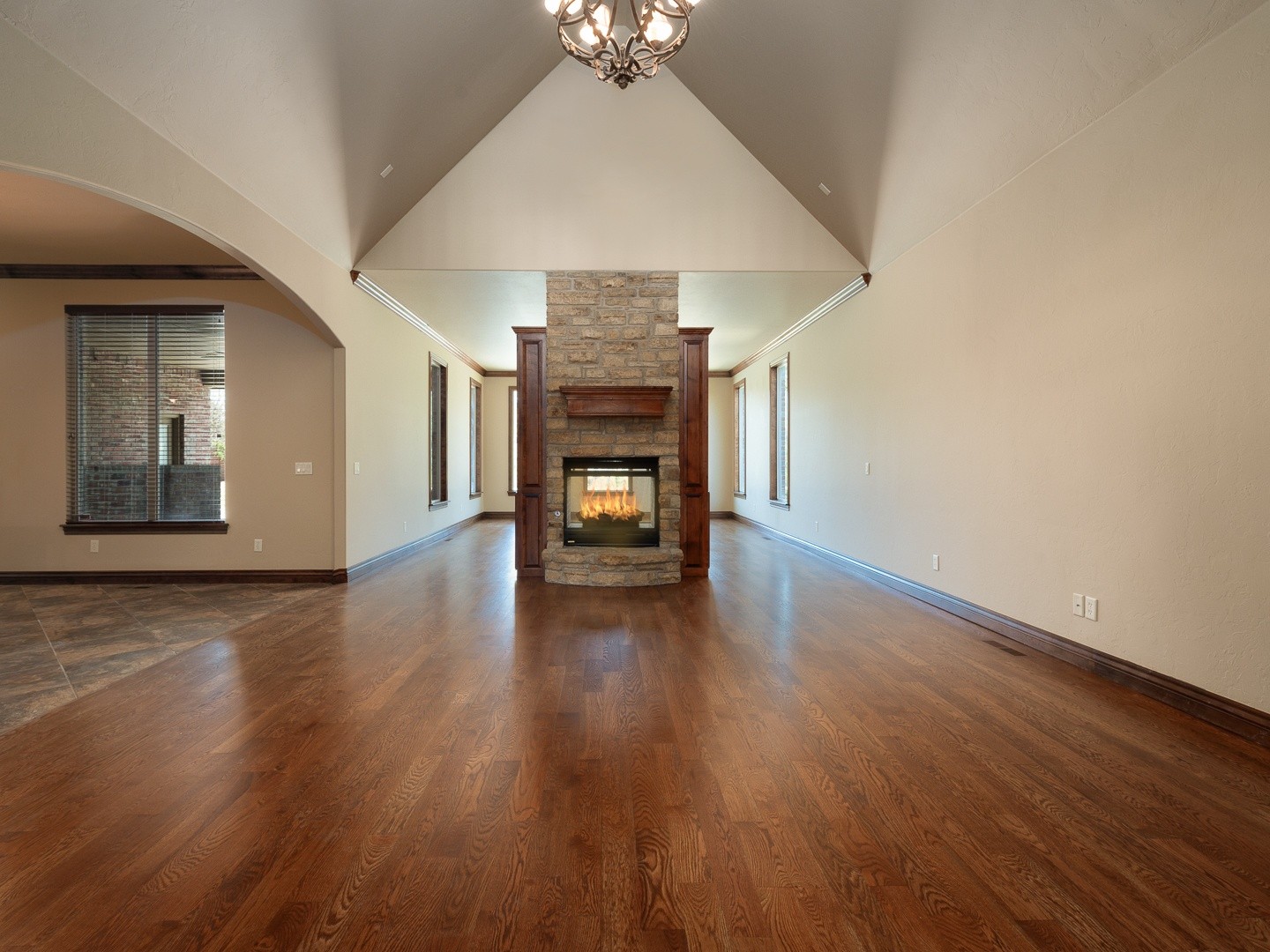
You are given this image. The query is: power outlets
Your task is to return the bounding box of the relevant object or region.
[254,539,263,552]
[933,555,939,571]
[1085,596,1098,621]
[90,540,99,553]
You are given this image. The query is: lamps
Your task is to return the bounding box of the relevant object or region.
[545,0,700,90]
